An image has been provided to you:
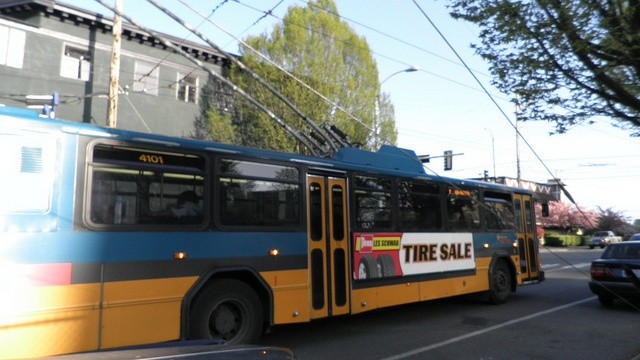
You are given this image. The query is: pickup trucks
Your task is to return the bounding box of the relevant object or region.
[590,231,622,249]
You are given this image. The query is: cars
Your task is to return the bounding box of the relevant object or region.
[32,339,296,360]
[628,233,640,241]
[589,241,640,306]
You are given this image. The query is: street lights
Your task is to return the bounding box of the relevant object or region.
[374,67,419,150]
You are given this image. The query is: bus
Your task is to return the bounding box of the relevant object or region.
[0,96,546,360]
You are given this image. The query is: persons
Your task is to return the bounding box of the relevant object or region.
[166,189,203,226]
